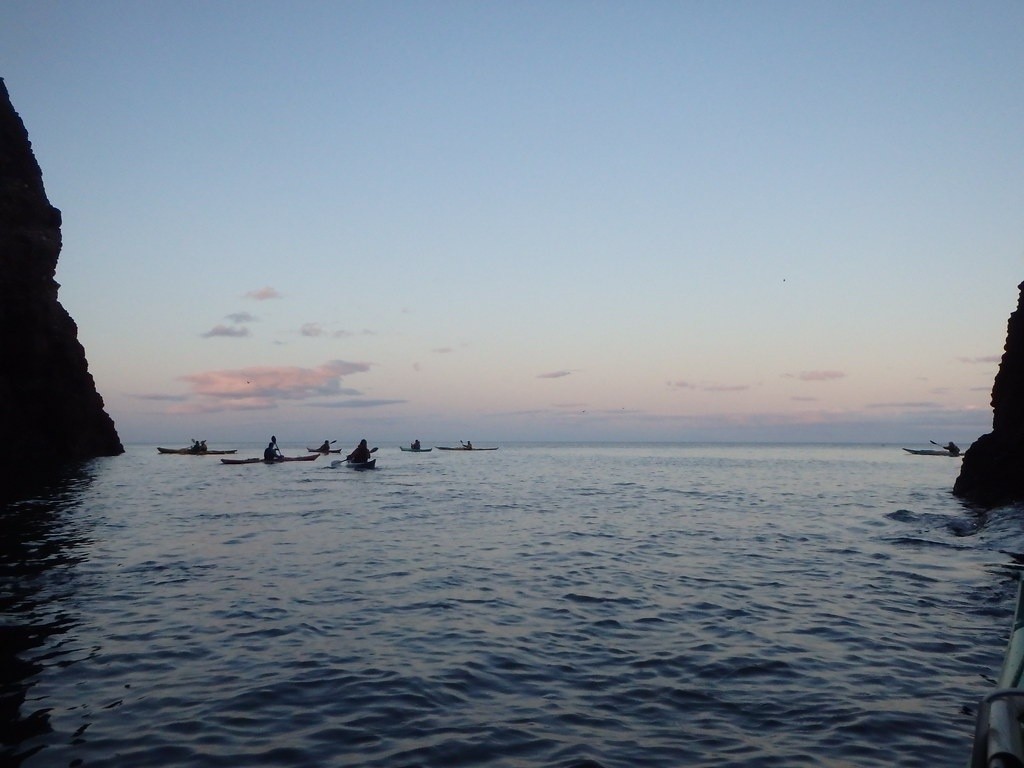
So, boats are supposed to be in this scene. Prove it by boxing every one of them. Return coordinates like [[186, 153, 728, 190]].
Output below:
[[400, 445, 432, 452], [345, 458, 376, 470], [221, 453, 321, 463], [902, 447, 965, 457], [157, 446, 237, 454], [306, 447, 342, 453], [434, 445, 500, 451]]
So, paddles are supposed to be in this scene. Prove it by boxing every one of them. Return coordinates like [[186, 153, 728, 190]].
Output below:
[[271, 435, 283, 462], [331, 447, 379, 467], [930, 440, 944, 448], [460, 440, 464, 445], [191, 439, 196, 445], [329, 439, 337, 445]]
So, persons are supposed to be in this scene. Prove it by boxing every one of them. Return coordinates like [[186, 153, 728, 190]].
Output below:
[[264, 442, 283, 461], [189, 441, 207, 452], [411, 440, 420, 450], [463, 441, 472, 450], [943, 441, 960, 454], [346, 438, 371, 463], [316, 440, 330, 452]]
